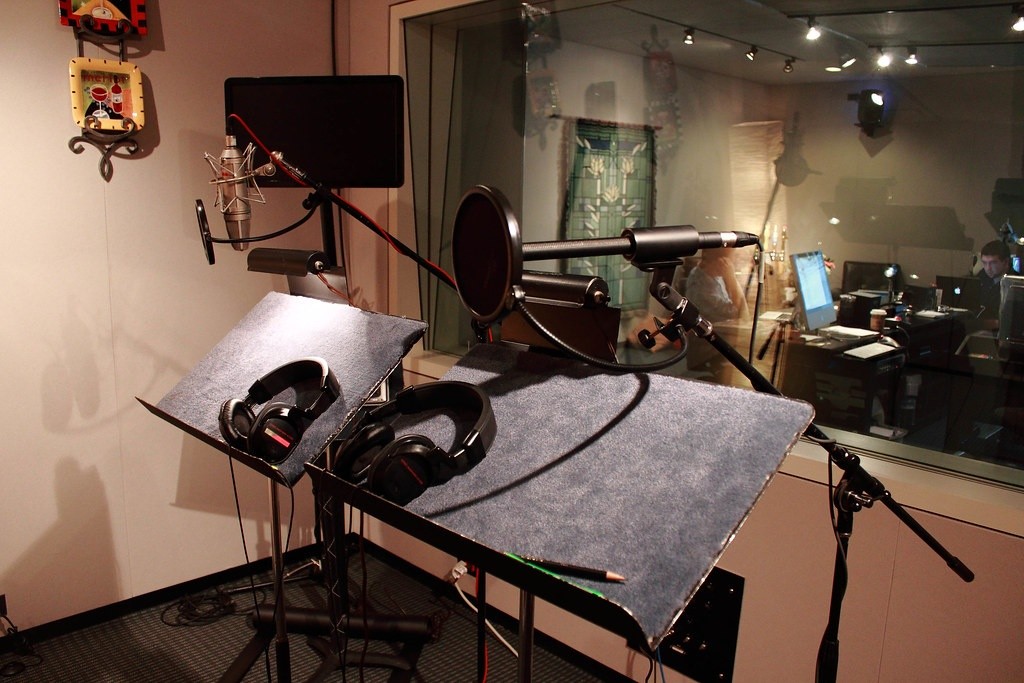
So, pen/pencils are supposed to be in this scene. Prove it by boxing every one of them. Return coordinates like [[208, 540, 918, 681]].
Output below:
[[504, 553, 627, 583], [830, 336, 843, 342]]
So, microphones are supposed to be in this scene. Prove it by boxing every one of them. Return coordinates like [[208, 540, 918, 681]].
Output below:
[[219, 125, 252, 252]]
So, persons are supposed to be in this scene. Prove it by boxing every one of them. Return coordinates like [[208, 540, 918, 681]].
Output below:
[[966, 241, 1021, 324], [686, 248, 749, 321]]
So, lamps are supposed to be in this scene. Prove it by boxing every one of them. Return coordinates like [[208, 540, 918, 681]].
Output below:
[[905, 47, 918, 65], [1013, 3, 1024, 32], [745, 45, 758, 62], [848, 89, 885, 138], [783, 57, 796, 73], [838, 43, 856, 68], [805, 15, 821, 41], [683, 27, 695, 45]]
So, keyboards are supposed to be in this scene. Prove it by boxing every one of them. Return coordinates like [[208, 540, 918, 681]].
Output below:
[[844, 342, 895, 359]]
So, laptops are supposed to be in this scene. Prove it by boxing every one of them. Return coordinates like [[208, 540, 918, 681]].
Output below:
[[935, 275, 983, 309]]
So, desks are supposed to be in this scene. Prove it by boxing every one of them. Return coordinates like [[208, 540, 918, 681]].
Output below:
[[685, 290, 992, 435]]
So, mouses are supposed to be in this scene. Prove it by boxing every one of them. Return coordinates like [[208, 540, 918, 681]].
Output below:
[[877, 335, 901, 347]]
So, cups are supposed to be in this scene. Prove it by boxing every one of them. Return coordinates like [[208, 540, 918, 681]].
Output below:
[[870, 309, 887, 332], [936, 289, 943, 307]]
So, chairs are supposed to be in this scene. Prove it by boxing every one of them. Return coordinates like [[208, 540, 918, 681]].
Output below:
[[841, 260, 905, 295]]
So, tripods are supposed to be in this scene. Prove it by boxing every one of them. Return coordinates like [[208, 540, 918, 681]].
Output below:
[[305, 496, 413, 683]]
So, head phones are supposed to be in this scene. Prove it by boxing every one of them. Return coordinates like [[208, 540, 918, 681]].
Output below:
[[334, 380, 497, 505], [218, 357, 341, 465]]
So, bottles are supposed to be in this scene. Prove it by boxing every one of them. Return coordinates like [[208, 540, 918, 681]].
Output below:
[[904, 304, 913, 323]]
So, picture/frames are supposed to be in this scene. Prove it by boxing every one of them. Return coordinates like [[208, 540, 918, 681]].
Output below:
[[58, 0, 148, 37], [70, 56, 145, 132]]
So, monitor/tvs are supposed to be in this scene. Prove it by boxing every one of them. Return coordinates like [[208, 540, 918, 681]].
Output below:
[[789, 250, 836, 331]]
